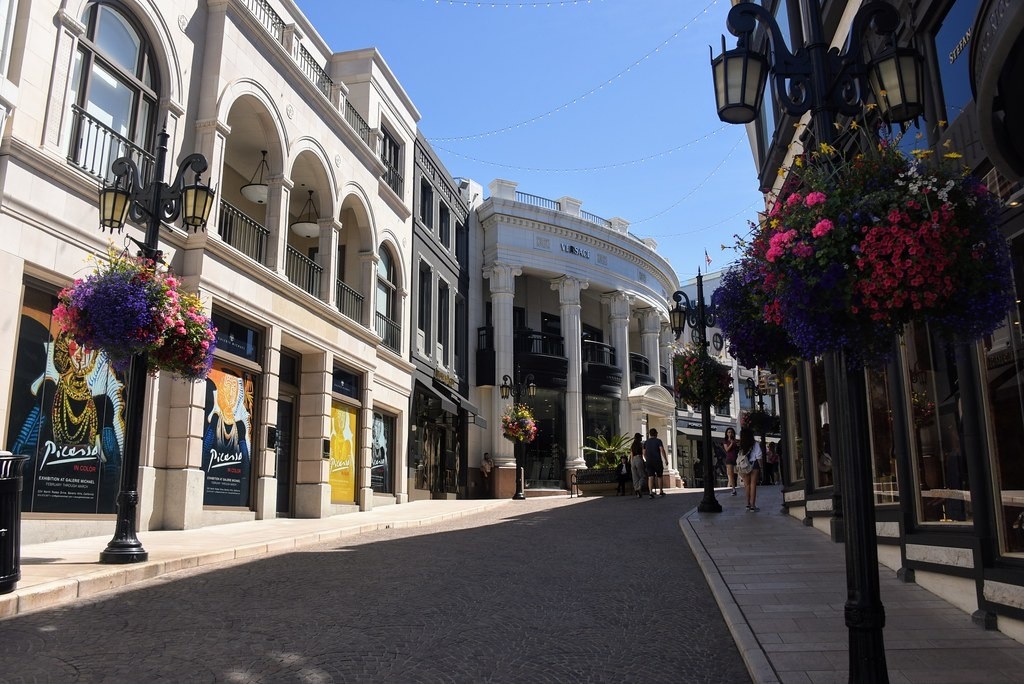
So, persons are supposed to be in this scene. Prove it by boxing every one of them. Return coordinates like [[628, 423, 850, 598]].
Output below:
[[643, 428, 668, 499], [820, 424, 832, 484], [740, 428, 763, 512], [722, 427, 740, 496], [616, 455, 632, 496], [767, 440, 782, 485], [480, 452, 494, 498], [631, 432, 645, 498]]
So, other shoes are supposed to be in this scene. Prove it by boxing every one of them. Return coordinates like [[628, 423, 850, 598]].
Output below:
[[635, 489, 641, 498], [745, 505, 761, 513], [649, 494, 654, 499], [659, 492, 666, 497], [731, 492, 736, 496], [621, 493, 625, 496]]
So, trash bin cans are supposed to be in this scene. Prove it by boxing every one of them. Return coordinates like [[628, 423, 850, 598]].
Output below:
[[0, 454, 31, 595]]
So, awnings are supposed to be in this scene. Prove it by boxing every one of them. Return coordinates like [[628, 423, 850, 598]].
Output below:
[[677, 427, 780, 443], [417, 379, 487, 429]]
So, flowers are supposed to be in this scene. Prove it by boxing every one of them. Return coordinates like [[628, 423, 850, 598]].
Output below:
[[52, 242, 217, 381], [708, 88, 1019, 375], [667, 342, 735, 410], [502, 405, 538, 444], [909, 390, 935, 428], [740, 410, 772, 435]]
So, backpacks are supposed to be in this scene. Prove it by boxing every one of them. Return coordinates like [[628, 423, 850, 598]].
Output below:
[[736, 445, 753, 475]]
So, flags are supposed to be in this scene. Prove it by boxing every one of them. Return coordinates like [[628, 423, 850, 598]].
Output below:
[[705, 251, 712, 265]]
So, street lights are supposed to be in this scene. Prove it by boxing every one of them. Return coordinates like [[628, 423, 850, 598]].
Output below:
[[668, 265, 732, 513], [96, 115, 218, 565], [500, 362, 537, 500], [707, 0, 929, 684], [745, 377, 776, 485]]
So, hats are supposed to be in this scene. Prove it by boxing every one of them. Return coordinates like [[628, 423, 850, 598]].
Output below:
[[634, 433, 643, 437], [620, 455, 628, 461]]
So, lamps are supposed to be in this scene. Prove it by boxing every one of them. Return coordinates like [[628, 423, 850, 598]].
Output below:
[[290, 190, 321, 239], [239, 150, 272, 204]]
